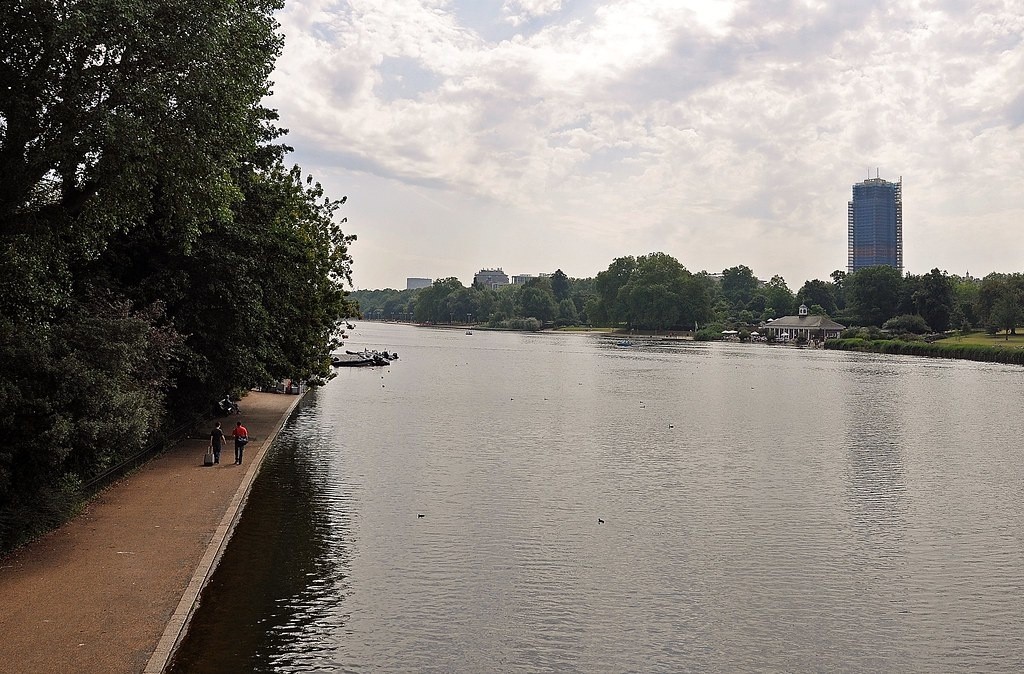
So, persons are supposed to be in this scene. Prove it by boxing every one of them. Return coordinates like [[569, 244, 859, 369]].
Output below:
[[233, 422, 248, 465], [225, 395, 242, 415], [211, 422, 226, 464]]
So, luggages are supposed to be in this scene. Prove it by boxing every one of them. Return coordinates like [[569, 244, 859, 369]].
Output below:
[[204, 445, 214, 466]]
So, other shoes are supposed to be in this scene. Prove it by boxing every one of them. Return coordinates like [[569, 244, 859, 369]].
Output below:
[[214, 461, 219, 464], [235, 461, 238, 464], [239, 462, 241, 465]]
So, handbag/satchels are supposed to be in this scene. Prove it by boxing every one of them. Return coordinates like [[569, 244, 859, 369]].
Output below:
[[235, 436, 248, 446]]
